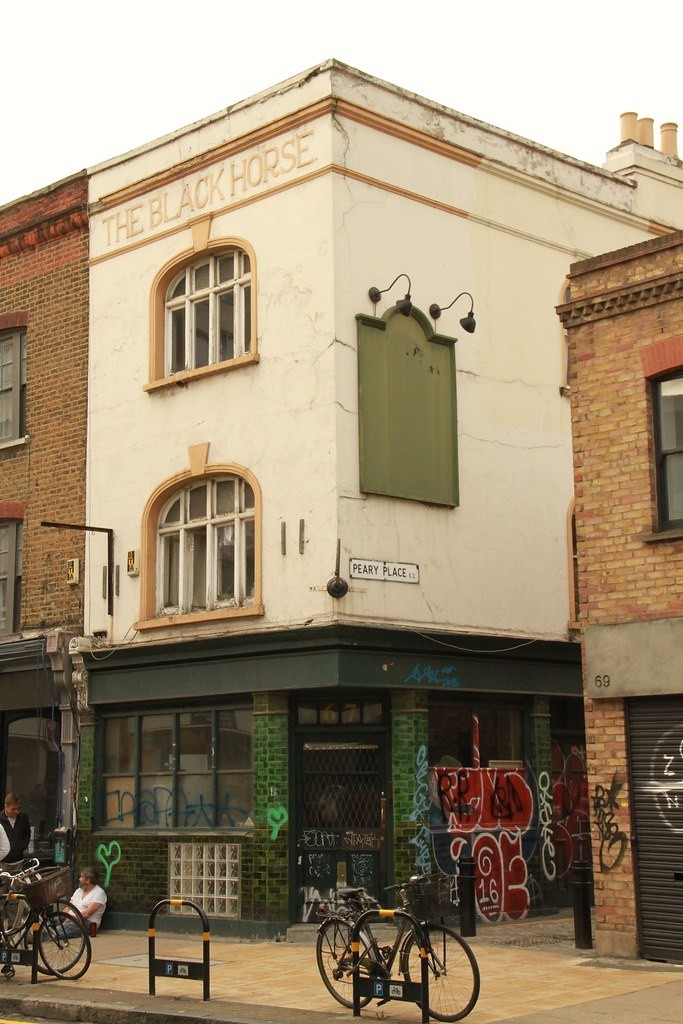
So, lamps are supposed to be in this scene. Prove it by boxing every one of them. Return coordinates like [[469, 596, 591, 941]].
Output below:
[[329, 538, 348, 599], [374, 298, 375, 299], [369, 273, 412, 317], [429, 292, 475, 334]]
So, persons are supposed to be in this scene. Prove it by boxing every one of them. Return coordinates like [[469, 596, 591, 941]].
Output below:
[[21, 869, 107, 944], [0, 793, 31, 931], [0, 824, 10, 860]]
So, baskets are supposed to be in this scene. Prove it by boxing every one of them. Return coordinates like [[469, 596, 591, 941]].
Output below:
[[405, 873, 452, 920], [18, 866, 73, 910]]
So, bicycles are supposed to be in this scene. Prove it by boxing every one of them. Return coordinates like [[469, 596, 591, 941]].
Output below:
[[1, 858, 92, 981], [300, 870, 482, 1024]]
